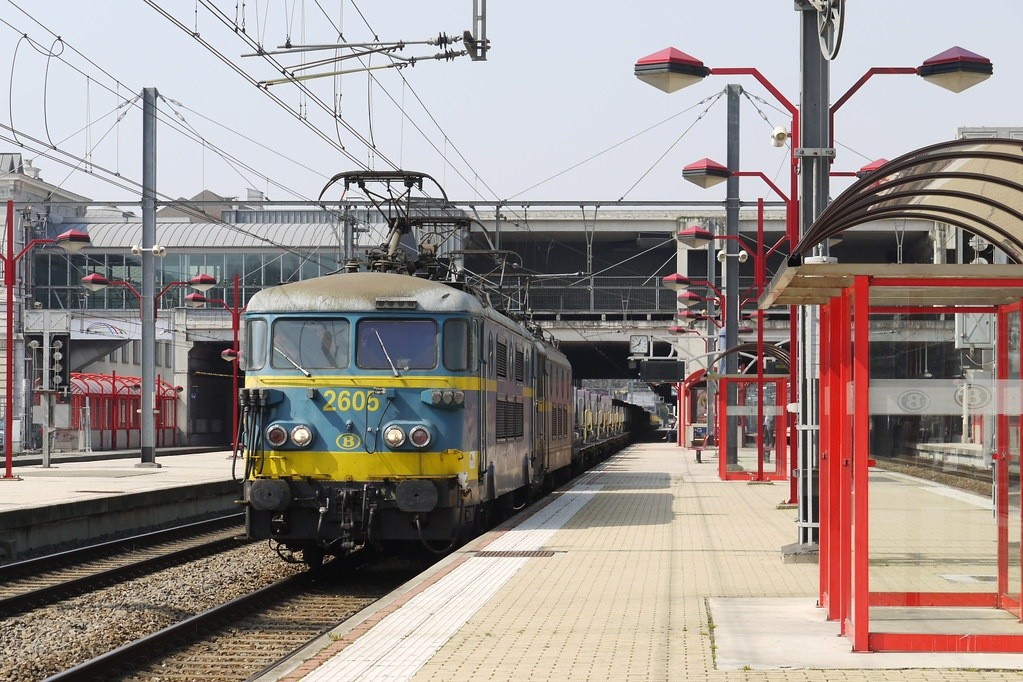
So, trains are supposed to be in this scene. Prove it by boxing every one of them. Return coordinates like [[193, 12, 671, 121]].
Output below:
[[232, 264, 660, 578]]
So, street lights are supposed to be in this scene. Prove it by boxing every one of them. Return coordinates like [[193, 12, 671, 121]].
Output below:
[[631, 42, 995, 182], [187, 288, 245, 461], [679, 155, 894, 256], [664, 227, 801, 487], [80, 265, 216, 320], [0, 198, 90, 479]]
